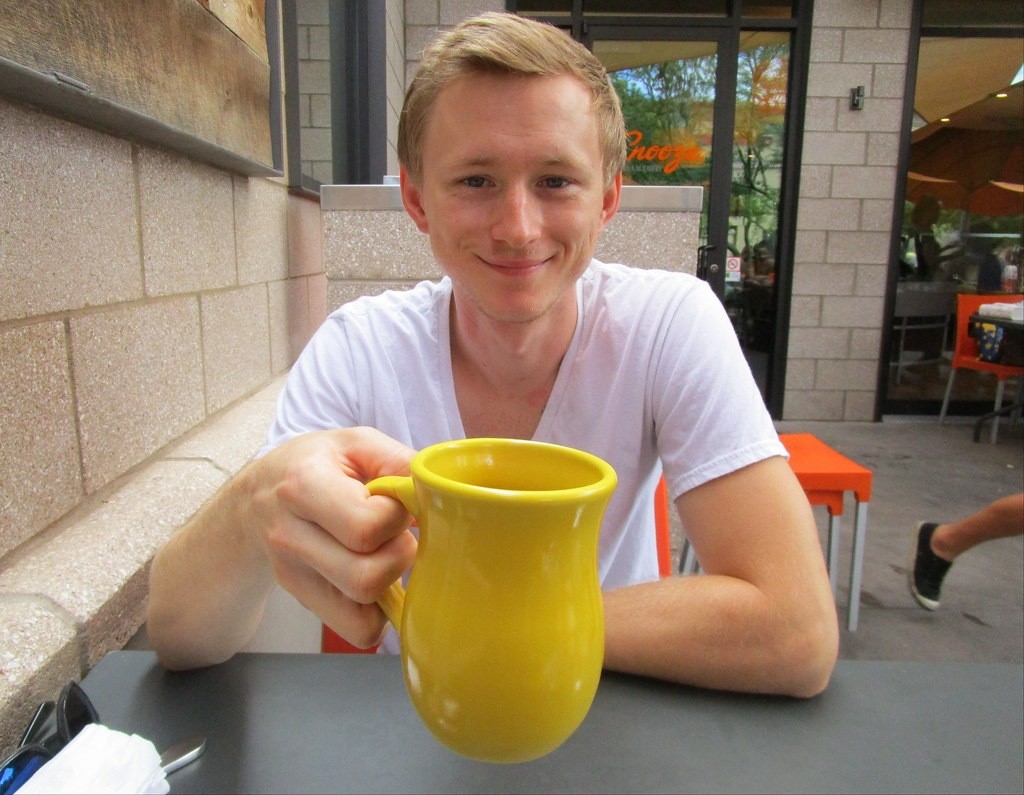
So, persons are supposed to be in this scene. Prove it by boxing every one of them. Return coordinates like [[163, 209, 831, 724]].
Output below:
[[743, 195, 1024, 360], [907, 491, 1024, 611], [146, 9, 841, 697]]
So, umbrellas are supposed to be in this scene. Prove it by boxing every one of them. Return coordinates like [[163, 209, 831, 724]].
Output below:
[[903, 80, 1024, 217]]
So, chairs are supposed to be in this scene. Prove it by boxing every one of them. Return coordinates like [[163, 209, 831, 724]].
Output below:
[[938, 293, 1024, 445]]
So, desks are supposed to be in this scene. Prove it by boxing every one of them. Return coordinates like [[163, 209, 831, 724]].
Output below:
[[968, 312, 1024, 441], [889, 283, 952, 387], [79, 650, 1024, 795]]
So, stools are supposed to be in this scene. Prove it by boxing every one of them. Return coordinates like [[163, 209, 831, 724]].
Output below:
[[678, 433, 871, 632]]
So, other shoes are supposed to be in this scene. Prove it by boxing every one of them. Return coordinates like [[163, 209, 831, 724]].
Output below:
[[911, 520, 953, 610]]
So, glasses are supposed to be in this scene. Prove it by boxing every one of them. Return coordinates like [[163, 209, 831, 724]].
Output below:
[[0, 681, 101, 795]]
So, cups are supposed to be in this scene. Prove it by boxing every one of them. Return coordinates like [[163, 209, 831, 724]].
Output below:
[[1000, 264, 1018, 295], [365, 438, 618, 765]]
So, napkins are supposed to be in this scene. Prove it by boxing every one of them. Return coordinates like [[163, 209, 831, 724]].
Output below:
[[10, 722, 171, 795]]
